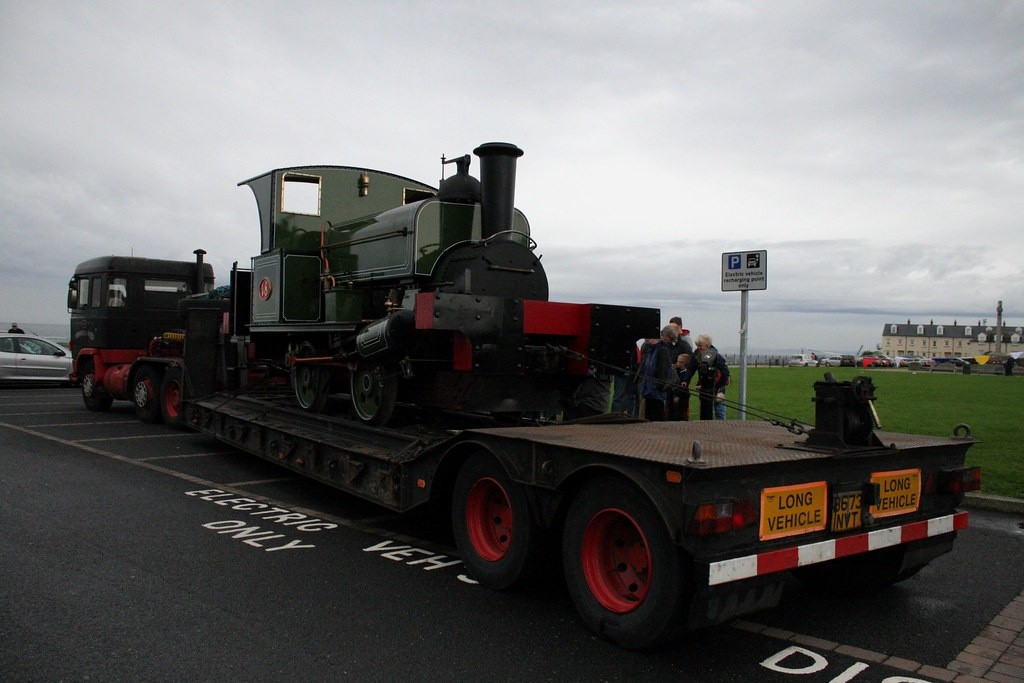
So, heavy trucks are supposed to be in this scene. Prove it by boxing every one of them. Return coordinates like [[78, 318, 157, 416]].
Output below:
[[68, 256, 985, 653]]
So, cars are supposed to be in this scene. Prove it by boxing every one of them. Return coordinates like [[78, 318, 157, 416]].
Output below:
[[931, 357, 970, 368], [821, 356, 841, 367], [860, 354, 894, 367], [840, 355, 859, 367], [0, 332, 73, 384], [899, 355, 930, 367], [788, 354, 820, 367]]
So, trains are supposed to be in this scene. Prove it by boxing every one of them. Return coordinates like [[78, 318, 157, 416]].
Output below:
[[177, 142, 661, 426]]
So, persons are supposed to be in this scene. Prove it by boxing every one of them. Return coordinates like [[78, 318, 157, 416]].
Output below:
[[573, 316, 731, 421], [7, 323, 25, 347]]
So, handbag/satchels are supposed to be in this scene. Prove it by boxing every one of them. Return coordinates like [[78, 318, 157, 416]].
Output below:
[[714, 357, 730, 386]]
